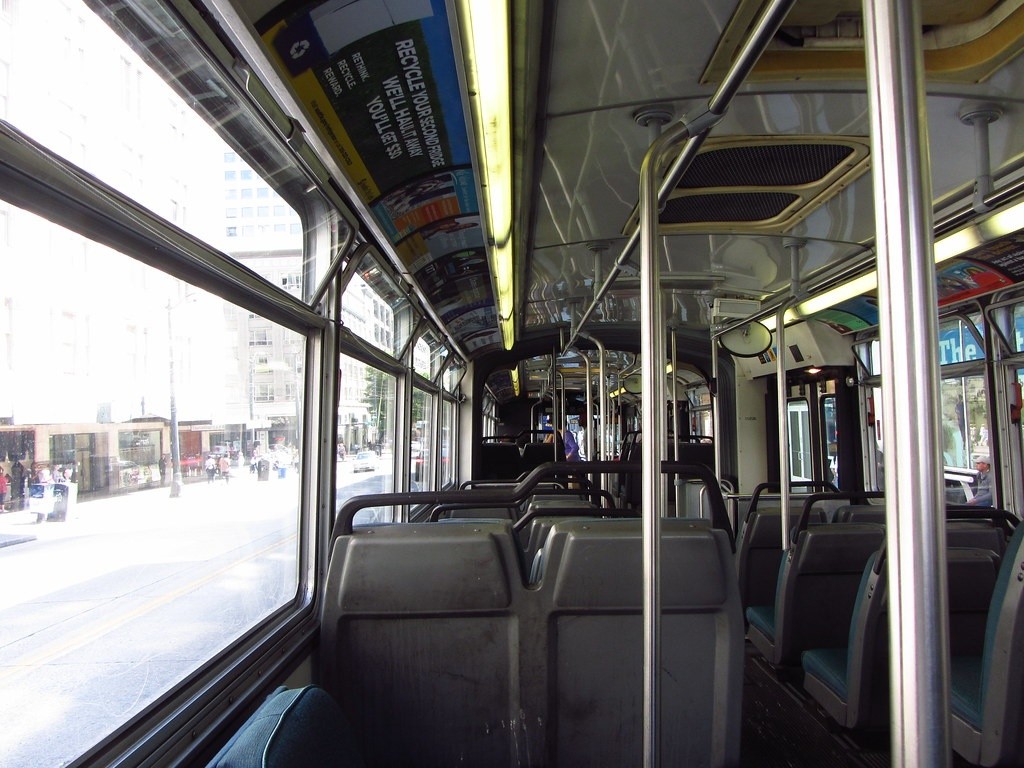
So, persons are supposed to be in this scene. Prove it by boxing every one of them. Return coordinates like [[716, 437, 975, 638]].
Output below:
[[249, 440, 345, 473], [355, 443, 358, 454], [965, 456, 992, 507], [158, 450, 230, 484], [501, 417, 581, 490], [0, 455, 27, 513]]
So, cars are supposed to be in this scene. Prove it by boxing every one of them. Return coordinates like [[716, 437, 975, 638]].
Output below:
[[415, 448, 448, 480], [353, 451, 378, 472]]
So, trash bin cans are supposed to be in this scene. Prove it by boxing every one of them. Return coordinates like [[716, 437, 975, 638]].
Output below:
[[258, 460, 270, 481], [48, 483, 78, 522], [374, 445, 382, 456]]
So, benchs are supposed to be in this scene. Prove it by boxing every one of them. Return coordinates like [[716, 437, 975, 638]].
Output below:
[[204, 429, 1024, 768]]
